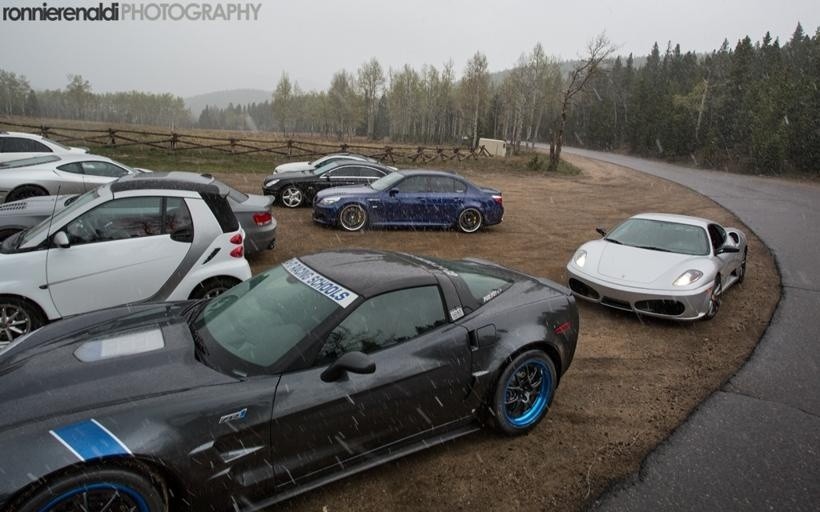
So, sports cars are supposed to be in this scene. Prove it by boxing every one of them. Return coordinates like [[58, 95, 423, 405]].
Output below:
[[1, 249, 579, 511], [567, 212, 749, 322]]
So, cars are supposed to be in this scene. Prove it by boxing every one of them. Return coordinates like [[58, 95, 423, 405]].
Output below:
[[274, 153, 378, 174], [1, 170, 278, 257], [313, 170, 505, 233], [0, 153, 147, 204], [0, 181, 252, 344], [260, 162, 400, 207], [0, 130, 90, 164]]
